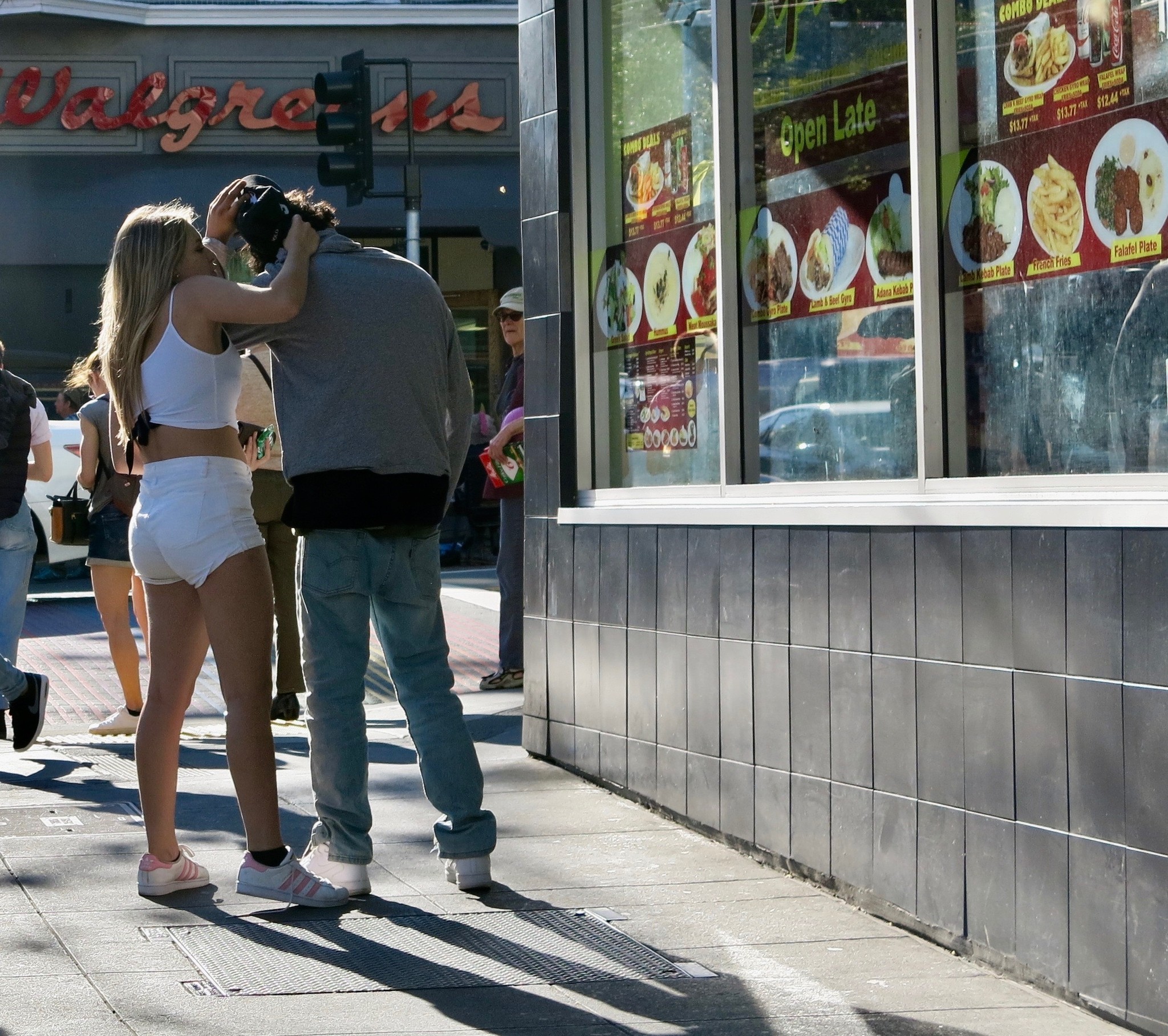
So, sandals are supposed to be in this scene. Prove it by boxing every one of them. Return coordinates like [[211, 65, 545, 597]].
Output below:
[[479, 666, 523, 690]]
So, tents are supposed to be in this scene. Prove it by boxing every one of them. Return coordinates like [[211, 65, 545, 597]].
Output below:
[[479, 441, 525, 488]]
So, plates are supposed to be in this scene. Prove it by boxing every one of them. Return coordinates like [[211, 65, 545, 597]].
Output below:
[[685, 380, 693, 398], [1027, 163, 1084, 258], [1085, 118, 1168, 250], [661, 428, 679, 447], [865, 193, 913, 285], [688, 400, 696, 418], [688, 420, 696, 447], [596, 266, 642, 339], [742, 221, 798, 310], [682, 230, 717, 319], [948, 160, 1023, 273], [640, 407, 660, 423], [799, 224, 865, 300], [679, 429, 688, 447], [644, 242, 681, 331], [644, 429, 661, 448]]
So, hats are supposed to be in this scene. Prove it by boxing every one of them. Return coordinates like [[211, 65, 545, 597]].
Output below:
[[63, 387, 83, 408], [235, 174, 292, 254], [491, 286, 523, 313]]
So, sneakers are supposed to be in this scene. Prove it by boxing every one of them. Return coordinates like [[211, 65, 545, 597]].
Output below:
[[299, 839, 370, 895], [444, 854, 491, 889], [8, 672, 49, 752], [138, 843, 210, 895], [234, 845, 350, 907], [89, 704, 140, 736]]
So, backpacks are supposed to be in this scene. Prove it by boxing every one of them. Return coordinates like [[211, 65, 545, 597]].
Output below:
[[96, 398, 142, 516]]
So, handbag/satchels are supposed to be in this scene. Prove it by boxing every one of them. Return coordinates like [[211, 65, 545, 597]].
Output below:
[[47, 481, 92, 546]]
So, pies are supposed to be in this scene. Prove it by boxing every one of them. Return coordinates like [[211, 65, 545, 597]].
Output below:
[[1010, 29, 1039, 74], [628, 163, 645, 195], [805, 233, 834, 296]]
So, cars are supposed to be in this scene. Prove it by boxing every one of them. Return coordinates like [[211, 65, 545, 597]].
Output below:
[[752, 356, 923, 482], [26, 420, 101, 566]]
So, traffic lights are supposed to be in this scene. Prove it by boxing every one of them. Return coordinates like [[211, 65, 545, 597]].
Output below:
[[315, 48, 372, 208]]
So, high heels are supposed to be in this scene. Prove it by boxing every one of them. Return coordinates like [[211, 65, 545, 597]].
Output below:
[[270, 692, 300, 720]]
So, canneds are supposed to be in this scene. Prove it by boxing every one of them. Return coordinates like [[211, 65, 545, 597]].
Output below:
[[663, 137, 688, 195], [1076, 0, 1124, 68]]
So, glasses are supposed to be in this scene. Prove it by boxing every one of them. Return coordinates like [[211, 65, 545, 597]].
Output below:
[[495, 311, 523, 322], [87, 386, 95, 399]]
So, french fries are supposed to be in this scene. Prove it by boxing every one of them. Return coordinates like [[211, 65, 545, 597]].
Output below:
[[638, 161, 661, 203], [1033, 154, 1080, 257], [1032, 25, 1070, 84]]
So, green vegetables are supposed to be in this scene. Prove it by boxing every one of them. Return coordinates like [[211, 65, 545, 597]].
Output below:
[[749, 234, 767, 272], [694, 240, 715, 283], [653, 250, 670, 304], [869, 202, 902, 257], [963, 163, 1009, 225], [603, 252, 636, 331], [1094, 156, 1121, 231]]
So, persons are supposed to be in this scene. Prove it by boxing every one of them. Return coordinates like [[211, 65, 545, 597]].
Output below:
[[0, 343, 54, 750], [65, 353, 151, 734], [55, 388, 83, 421], [93, 206, 348, 908], [201, 175, 496, 895], [1111, 262, 1168, 473], [234, 344, 305, 722], [480, 288, 524, 688]]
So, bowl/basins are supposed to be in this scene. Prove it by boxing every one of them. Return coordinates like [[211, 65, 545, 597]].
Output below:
[[1004, 31, 1076, 97], [626, 167, 664, 212], [660, 412, 670, 422]]
